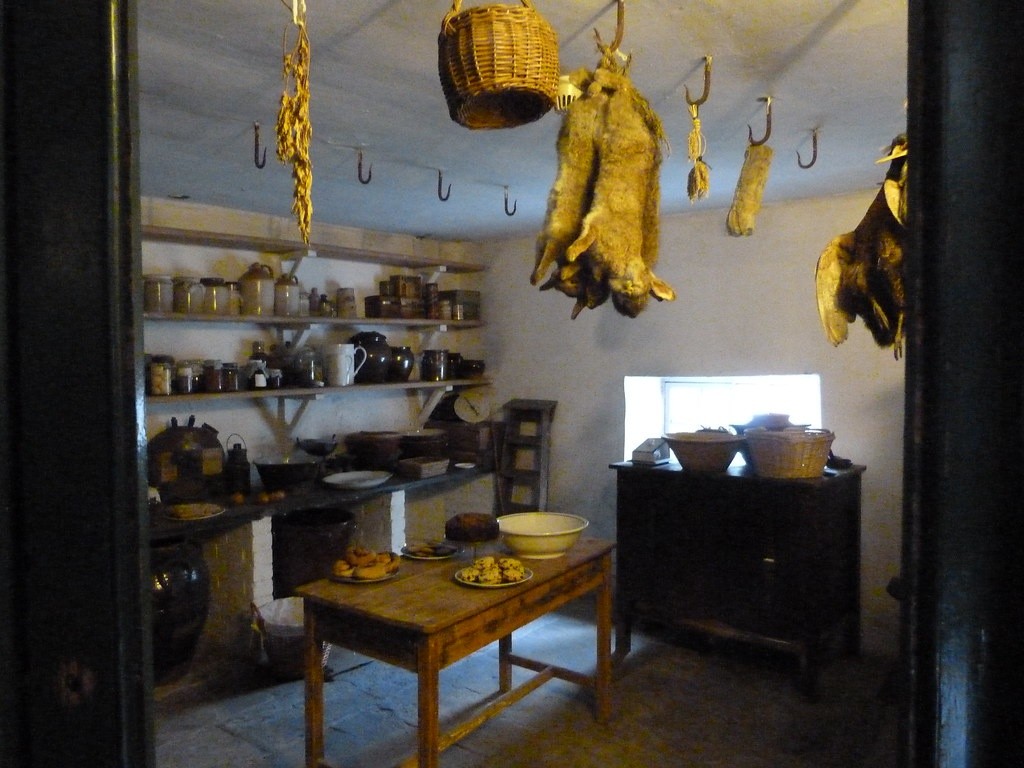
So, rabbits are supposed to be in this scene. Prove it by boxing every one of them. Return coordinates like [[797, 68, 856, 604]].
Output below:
[[528, 47, 677, 324]]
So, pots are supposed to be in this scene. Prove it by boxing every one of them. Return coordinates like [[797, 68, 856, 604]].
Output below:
[[252, 452, 319, 494]]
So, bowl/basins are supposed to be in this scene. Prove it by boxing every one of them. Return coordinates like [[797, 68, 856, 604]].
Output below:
[[498, 512, 590, 559], [402, 429, 450, 458], [345, 430, 400, 467], [463, 359, 483, 377], [662, 432, 745, 472]]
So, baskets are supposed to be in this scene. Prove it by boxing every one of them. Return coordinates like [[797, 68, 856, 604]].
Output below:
[[436, 0, 559, 130], [743, 427, 835, 478], [398, 456, 449, 479]]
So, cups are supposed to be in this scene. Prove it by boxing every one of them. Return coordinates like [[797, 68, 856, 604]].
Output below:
[[322, 341, 367, 387]]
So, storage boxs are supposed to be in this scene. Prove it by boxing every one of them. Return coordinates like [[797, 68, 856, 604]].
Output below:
[[365, 275, 482, 320]]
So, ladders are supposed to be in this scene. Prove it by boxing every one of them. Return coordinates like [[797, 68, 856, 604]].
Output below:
[[500, 399, 558, 513]]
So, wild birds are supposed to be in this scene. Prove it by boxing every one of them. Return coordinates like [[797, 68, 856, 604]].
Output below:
[[817, 134, 908, 361]]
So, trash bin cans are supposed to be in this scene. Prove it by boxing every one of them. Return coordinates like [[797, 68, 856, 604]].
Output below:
[[273, 508, 356, 599]]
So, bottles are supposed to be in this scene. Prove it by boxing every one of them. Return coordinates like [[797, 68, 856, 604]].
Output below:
[[148, 539, 210, 686], [145, 262, 333, 318], [349, 331, 391, 383], [226, 444, 251, 495], [423, 282, 464, 320], [145, 339, 319, 396], [421, 349, 449, 381], [446, 352, 463, 378], [388, 346, 414, 382]]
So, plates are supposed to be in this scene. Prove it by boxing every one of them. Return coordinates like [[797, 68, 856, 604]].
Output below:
[[455, 567, 533, 587], [401, 546, 457, 560], [328, 567, 401, 582], [455, 463, 475, 469], [322, 470, 394, 490], [161, 503, 226, 520]]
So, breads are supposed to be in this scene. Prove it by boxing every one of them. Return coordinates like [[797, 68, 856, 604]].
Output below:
[[334, 545, 401, 579], [458, 556, 524, 585]]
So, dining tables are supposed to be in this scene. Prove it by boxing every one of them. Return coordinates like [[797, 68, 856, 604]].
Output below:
[[295, 536, 618, 768]]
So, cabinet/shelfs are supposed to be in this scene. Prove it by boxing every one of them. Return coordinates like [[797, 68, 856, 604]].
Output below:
[[608, 456, 867, 706], [141, 223, 494, 449]]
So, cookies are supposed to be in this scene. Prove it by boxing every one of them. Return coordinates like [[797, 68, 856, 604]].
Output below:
[[405, 539, 459, 557]]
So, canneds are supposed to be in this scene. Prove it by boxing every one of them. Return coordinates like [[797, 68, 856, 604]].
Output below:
[[452, 303, 463, 321], [424, 282, 440, 319], [336, 287, 356, 319]]
